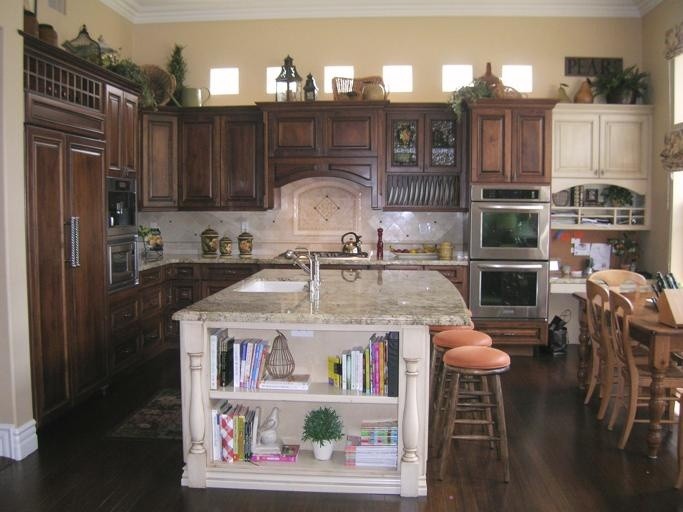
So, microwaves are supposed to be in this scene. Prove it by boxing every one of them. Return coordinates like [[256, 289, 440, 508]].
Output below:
[[104, 235, 137, 287], [107, 177, 138, 232]]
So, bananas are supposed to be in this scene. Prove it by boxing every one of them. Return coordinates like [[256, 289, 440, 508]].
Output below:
[[424, 244, 437, 253]]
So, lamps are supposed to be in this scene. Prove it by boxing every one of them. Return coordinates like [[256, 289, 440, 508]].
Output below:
[[302, 72, 319, 101], [273, 54, 305, 102]]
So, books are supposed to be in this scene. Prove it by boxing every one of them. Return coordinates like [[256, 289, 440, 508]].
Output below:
[[324, 330, 398, 396], [345, 417, 398, 473], [210, 326, 271, 391], [211, 400, 259, 463], [260, 374, 312, 391], [251, 444, 302, 462]]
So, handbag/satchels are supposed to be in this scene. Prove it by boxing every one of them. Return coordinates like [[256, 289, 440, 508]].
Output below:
[[548, 316, 569, 357]]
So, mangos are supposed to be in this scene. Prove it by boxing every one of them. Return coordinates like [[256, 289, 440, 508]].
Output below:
[[409, 249, 416, 253]]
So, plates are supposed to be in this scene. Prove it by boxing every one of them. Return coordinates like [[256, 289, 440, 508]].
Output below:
[[391, 251, 440, 257]]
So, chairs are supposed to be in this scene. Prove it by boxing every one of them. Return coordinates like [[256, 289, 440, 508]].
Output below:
[[609, 290, 683, 449], [587, 270, 650, 291], [583, 280, 650, 420]]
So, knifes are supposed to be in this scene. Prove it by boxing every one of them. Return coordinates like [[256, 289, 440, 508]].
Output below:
[[652, 271, 677, 297]]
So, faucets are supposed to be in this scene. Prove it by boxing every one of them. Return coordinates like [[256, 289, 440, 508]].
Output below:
[[293, 253, 321, 293]]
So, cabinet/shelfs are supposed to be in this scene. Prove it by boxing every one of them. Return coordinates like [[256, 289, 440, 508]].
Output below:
[[105, 83, 141, 180], [465, 98, 560, 184], [552, 103, 656, 178], [24, 121, 111, 432], [551, 178, 648, 294], [255, 101, 390, 210], [107, 263, 255, 369], [384, 264, 469, 322], [22, 34, 107, 140], [382, 103, 471, 211], [177, 320, 433, 499], [181, 106, 264, 211], [469, 320, 549, 357], [137, 107, 182, 211]]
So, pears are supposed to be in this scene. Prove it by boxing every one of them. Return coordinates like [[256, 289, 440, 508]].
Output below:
[[573, 82, 593, 104], [554, 86, 571, 102]]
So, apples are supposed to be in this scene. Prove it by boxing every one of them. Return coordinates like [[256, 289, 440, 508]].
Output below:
[[402, 249, 409, 253], [396, 248, 401, 253]]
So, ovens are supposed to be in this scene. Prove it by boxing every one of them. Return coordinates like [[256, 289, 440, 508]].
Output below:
[[469, 183, 550, 320]]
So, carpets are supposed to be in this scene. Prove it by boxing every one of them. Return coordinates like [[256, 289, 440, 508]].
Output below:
[[107, 385, 182, 441]]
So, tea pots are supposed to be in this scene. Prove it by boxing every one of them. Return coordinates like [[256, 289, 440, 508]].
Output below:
[[341, 232, 362, 254]]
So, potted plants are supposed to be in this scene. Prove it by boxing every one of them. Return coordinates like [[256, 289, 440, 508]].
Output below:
[[586, 60, 650, 104], [164, 44, 186, 106], [300, 404, 346, 462]]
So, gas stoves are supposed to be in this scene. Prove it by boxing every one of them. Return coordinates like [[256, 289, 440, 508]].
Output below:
[[274, 247, 372, 260]]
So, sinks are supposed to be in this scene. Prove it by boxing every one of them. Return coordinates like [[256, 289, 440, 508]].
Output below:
[[233, 280, 310, 294]]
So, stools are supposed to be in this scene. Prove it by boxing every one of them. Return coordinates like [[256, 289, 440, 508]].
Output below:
[[437, 346, 512, 482], [430, 324, 480, 411], [431, 330, 496, 450]]
